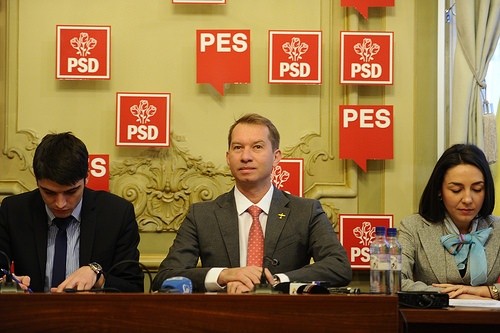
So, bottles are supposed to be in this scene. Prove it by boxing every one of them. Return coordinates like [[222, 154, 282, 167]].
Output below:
[[369, 226, 401, 293]]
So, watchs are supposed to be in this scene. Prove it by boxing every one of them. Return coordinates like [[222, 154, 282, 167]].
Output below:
[[87, 262, 102, 283]]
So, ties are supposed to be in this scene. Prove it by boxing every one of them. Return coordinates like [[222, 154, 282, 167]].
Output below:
[[246, 206, 264, 267], [50, 215, 74, 288]]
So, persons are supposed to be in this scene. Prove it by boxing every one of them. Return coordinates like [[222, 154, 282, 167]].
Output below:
[[0, 131, 140, 292], [380, 144, 500, 300], [152, 115, 351, 294]]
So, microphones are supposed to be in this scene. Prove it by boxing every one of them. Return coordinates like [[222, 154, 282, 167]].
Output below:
[[157, 276, 192, 294], [64, 287, 122, 293]]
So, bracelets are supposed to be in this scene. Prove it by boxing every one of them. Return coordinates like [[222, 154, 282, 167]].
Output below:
[[272, 275, 278, 287], [487, 286, 492, 298]]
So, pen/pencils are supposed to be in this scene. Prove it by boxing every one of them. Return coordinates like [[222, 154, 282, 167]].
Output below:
[[327, 287, 361, 293], [0, 269, 34, 294]]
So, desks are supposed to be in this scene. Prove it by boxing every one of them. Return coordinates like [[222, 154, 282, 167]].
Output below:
[[0, 292, 500, 333]]
